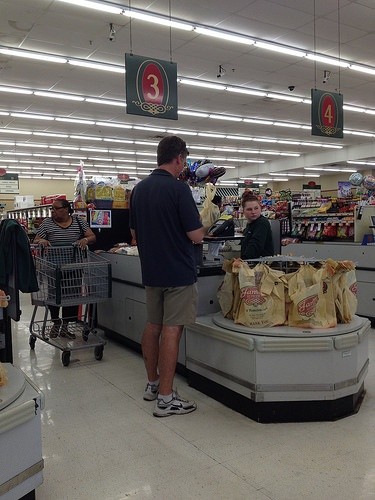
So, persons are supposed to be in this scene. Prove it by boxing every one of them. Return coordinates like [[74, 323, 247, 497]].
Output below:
[[129, 136, 205, 417], [33, 199, 97, 339], [211, 196, 222, 209], [238, 195, 274, 264]]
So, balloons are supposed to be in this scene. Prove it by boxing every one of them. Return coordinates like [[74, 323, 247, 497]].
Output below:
[[349, 172, 364, 187], [363, 175, 375, 191]]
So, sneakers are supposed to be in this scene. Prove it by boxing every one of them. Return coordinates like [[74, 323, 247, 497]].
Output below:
[[143, 383, 160, 401], [153, 390, 197, 417]]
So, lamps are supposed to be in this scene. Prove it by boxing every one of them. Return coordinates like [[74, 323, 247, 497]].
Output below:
[[0, 0, 375, 187]]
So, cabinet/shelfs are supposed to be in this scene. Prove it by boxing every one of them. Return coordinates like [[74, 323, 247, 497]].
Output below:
[[7, 201, 75, 243], [224, 196, 375, 244]]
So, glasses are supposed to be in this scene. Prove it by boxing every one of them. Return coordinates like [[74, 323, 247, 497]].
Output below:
[[50, 207, 67, 212]]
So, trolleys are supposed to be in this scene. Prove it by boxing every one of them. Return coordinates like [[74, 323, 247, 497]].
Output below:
[[29, 244, 112, 366]]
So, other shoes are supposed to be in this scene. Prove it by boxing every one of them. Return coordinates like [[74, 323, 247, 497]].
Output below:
[[50, 321, 61, 338], [60, 330, 76, 339]]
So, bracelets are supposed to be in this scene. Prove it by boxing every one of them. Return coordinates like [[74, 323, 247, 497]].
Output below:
[[84, 238, 88, 242]]
